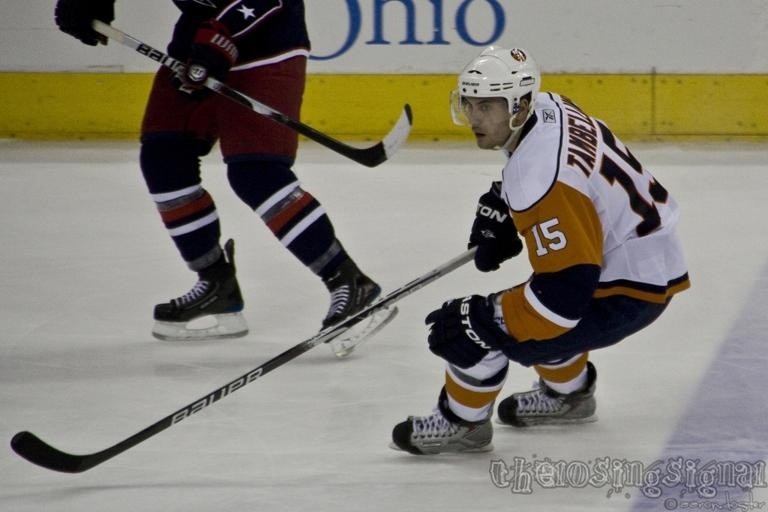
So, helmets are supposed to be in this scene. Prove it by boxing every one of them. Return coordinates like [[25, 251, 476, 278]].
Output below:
[[449, 45, 541, 132]]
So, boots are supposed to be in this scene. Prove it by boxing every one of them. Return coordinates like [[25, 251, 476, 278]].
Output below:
[[320, 238, 380, 343], [393, 385, 495, 456], [497, 361, 597, 427], [154, 238, 244, 322]]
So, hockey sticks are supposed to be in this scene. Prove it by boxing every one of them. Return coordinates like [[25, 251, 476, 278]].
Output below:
[[92, 18, 413, 167], [11, 246, 478, 474]]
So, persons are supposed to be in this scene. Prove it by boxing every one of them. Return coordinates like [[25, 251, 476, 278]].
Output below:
[[393, 45, 690, 455], [56, 1, 381, 345]]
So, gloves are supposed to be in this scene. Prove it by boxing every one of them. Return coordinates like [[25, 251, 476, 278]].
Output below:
[[55, 0, 115, 46], [425, 293, 519, 369], [467, 181, 523, 272], [168, 18, 240, 100]]
